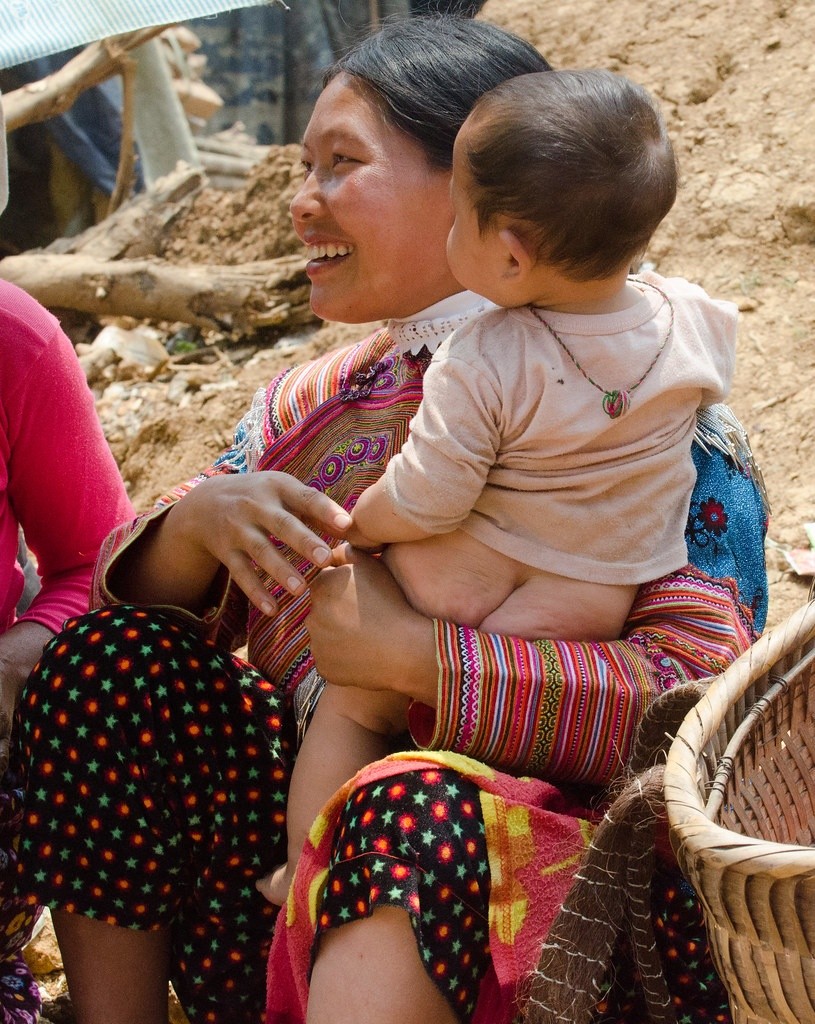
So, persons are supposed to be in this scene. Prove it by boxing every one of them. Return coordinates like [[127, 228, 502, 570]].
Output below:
[[256, 71, 741, 905], [0, 277, 141, 1024], [0, 20, 772, 1024]]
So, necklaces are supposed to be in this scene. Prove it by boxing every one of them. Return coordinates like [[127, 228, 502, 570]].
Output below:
[[527, 276, 674, 420]]
